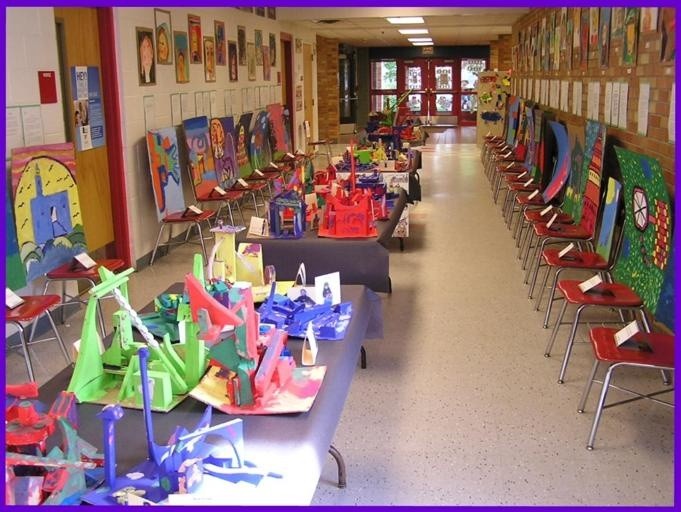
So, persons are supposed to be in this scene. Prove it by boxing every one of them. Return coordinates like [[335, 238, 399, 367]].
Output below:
[[230, 51, 238, 80], [204, 37, 215, 81], [178, 53, 188, 82], [140, 35, 156, 84]]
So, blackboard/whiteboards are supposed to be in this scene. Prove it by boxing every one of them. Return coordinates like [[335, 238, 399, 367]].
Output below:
[[4, 7, 68, 159]]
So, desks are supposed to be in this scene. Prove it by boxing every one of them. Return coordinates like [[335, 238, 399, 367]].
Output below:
[[367, 130, 430, 150], [235, 187, 413, 297], [6, 281, 384, 507], [332, 147, 422, 201]]
